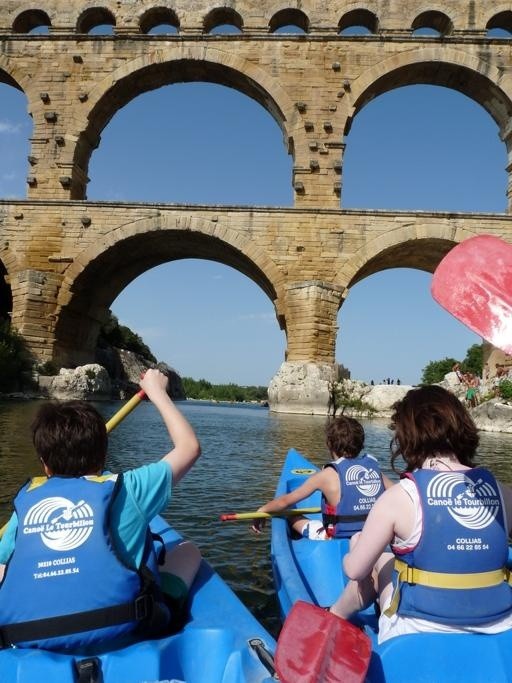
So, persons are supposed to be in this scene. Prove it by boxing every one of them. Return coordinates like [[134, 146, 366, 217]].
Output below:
[[251, 417, 396, 542], [328, 386, 512, 642], [0, 368, 201, 649], [369, 377, 402, 385], [483, 362, 509, 380], [451, 361, 481, 408]]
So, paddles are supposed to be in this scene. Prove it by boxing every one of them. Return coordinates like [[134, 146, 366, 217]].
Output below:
[[430, 234, 512, 358], [272, 599, 372, 683]]
